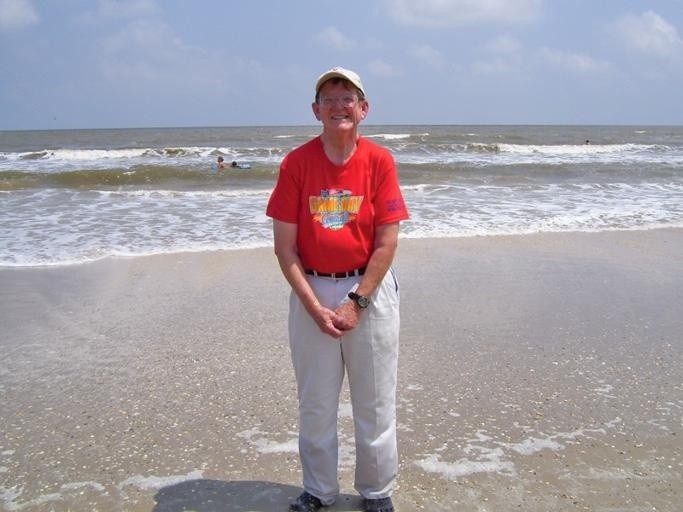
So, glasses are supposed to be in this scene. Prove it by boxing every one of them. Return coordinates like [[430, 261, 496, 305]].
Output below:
[[320, 95, 357, 107]]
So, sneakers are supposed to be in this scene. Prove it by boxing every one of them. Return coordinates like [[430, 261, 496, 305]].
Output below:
[[289, 492, 321, 512], [365, 497, 395, 511]]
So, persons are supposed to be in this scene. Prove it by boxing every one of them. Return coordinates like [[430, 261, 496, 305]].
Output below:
[[230, 161, 240, 168], [264, 66, 411, 512], [217, 156, 226, 168]]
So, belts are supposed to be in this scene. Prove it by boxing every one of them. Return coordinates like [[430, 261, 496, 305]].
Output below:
[[304, 268, 366, 279]]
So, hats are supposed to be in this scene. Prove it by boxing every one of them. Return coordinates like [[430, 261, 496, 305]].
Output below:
[[315, 66, 365, 95]]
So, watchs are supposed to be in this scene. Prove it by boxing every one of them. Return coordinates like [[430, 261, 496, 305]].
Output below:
[[348, 292, 371, 308]]
